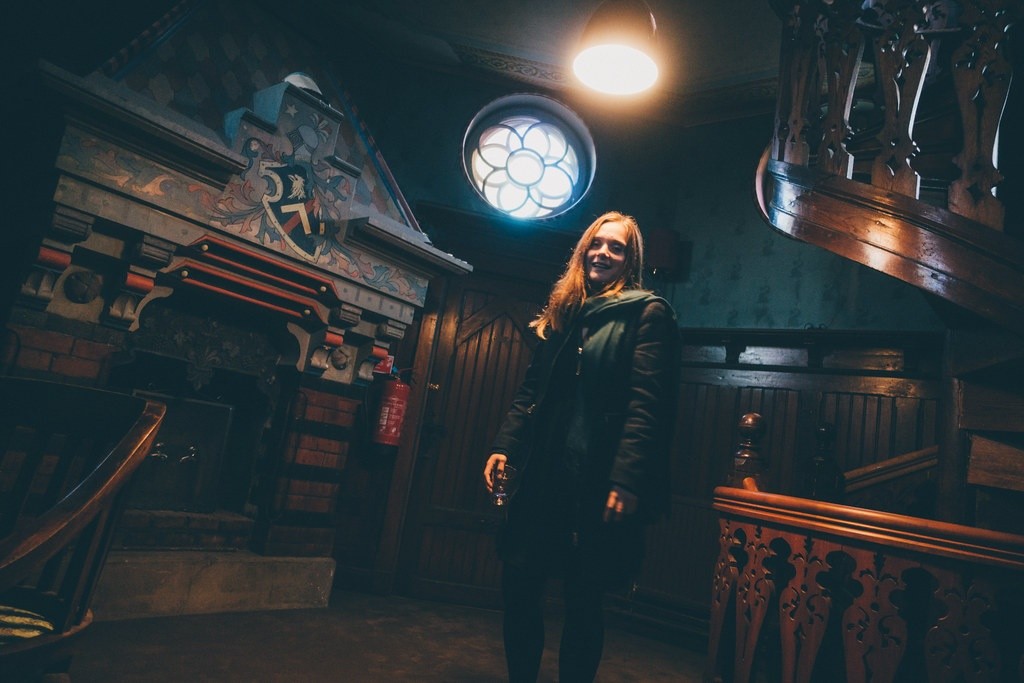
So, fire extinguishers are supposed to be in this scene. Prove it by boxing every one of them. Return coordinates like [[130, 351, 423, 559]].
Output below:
[[373, 366, 418, 448]]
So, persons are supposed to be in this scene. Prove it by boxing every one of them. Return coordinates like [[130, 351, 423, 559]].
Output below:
[[484, 212, 684, 683]]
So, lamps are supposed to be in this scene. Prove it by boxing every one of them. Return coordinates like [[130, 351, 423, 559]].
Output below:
[[573, 1, 665, 97]]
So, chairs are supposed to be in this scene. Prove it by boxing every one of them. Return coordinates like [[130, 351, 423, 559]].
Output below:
[[0, 370, 165, 683]]
